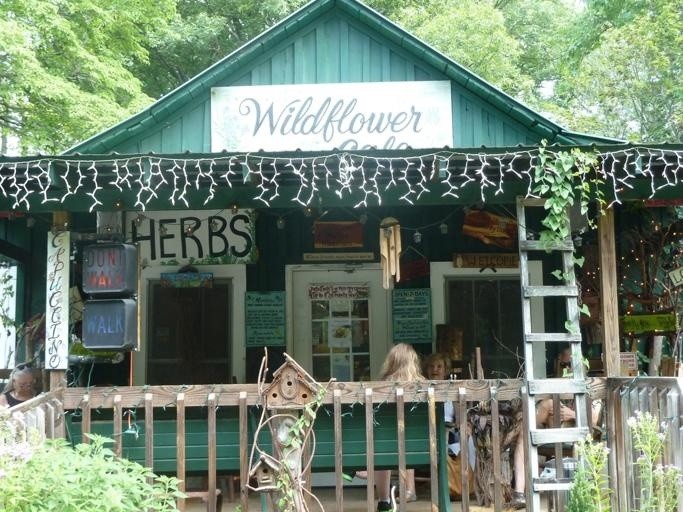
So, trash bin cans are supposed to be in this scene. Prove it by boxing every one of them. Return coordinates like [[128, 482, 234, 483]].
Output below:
[[542, 458, 581, 512]]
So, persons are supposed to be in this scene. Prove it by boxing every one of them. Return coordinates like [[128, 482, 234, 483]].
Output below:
[[496, 348, 602, 508], [0, 363, 44, 408], [340, 343, 424, 512], [392, 353, 473, 503]]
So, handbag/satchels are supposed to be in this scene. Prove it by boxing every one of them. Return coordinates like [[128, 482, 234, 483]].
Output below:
[[446, 448, 474, 496]]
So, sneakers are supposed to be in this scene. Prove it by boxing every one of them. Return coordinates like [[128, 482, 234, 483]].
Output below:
[[340, 469, 356, 481], [376, 499, 394, 512], [395, 491, 417, 503], [511, 491, 526, 508]]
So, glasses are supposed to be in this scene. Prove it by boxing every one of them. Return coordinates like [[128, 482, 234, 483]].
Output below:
[[16, 361, 33, 370], [559, 360, 572, 369]]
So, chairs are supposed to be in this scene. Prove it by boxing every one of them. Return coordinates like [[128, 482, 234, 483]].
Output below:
[[530, 365, 614, 471]]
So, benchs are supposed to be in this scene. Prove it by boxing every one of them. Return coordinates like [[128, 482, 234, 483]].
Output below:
[[66, 401, 453, 512]]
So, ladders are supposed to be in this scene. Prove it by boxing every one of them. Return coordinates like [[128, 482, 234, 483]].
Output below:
[[516, 194, 591, 512]]
